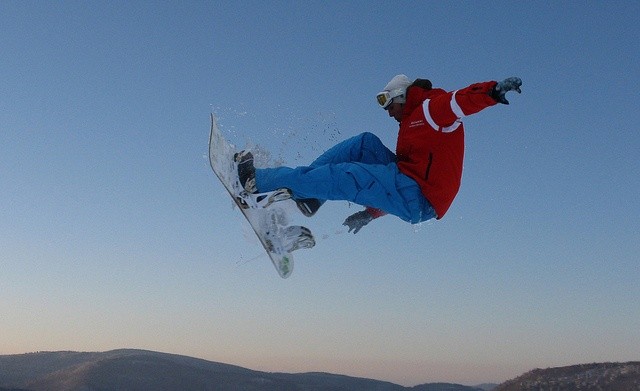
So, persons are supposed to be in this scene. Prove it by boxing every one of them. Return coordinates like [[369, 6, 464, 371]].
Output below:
[[233, 73, 524, 238]]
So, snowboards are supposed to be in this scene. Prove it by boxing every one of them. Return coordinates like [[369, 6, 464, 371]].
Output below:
[[208, 113, 295, 279]]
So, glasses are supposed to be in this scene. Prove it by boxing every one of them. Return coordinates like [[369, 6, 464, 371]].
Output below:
[[377, 88, 405, 108]]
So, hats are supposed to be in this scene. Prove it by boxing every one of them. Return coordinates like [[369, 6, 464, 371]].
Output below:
[[384, 75, 412, 103]]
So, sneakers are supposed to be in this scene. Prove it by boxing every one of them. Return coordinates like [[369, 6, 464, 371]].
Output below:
[[297, 198, 321, 217], [238, 150, 259, 194]]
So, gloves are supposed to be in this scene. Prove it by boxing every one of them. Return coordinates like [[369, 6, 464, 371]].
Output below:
[[491, 77, 522, 105], [342, 211, 373, 234]]
